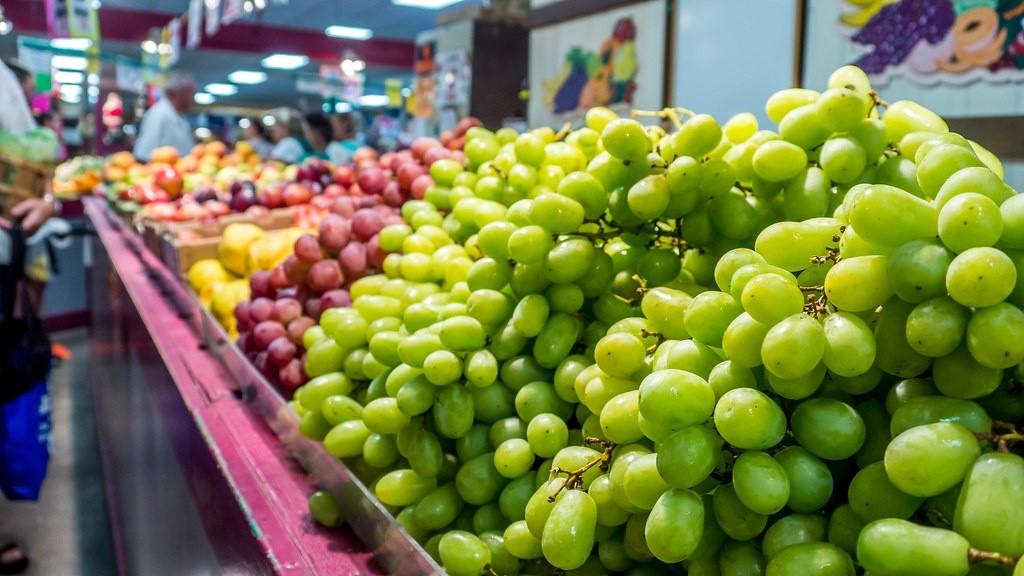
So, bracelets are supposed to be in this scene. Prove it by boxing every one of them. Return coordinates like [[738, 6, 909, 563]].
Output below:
[[44, 194, 64, 216]]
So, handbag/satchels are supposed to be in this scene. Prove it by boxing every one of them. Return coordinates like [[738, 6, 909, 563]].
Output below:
[[0, 215, 51, 501]]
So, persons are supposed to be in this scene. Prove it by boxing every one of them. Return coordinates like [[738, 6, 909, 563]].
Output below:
[[241, 107, 370, 169], [0, 58, 84, 575], [132, 72, 197, 165]]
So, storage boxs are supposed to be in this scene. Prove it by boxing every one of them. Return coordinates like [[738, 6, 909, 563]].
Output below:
[[146, 204, 295, 284]]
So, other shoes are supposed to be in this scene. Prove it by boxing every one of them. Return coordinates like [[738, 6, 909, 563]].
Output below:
[[0, 536, 29, 576]]
